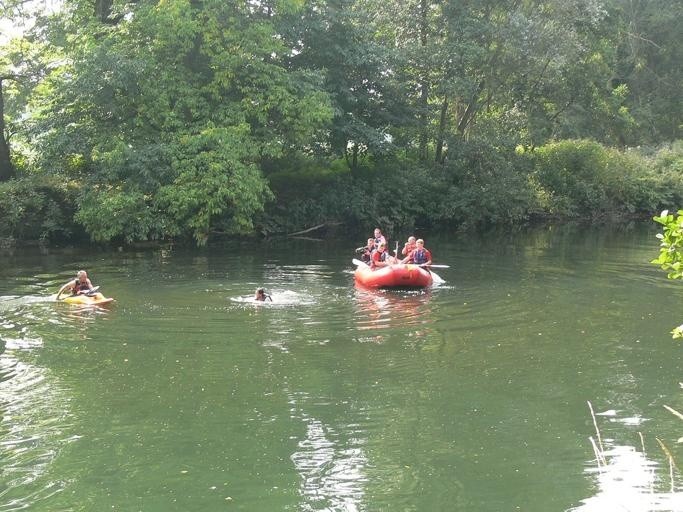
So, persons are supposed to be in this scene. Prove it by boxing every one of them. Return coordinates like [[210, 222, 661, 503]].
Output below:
[[56, 270, 93, 300], [402, 236, 417, 255], [370, 242, 390, 271], [361, 238, 375, 264], [400, 239, 432, 271], [254, 288, 272, 302], [373, 228, 386, 249]]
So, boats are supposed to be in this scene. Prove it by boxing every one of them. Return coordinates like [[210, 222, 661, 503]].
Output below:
[[353, 254, 434, 289], [352, 284, 432, 312], [56, 289, 115, 308]]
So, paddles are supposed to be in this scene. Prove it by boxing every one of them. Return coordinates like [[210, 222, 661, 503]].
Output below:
[[61, 286, 99, 300], [415, 264, 450, 268], [351, 259, 368, 267]]
